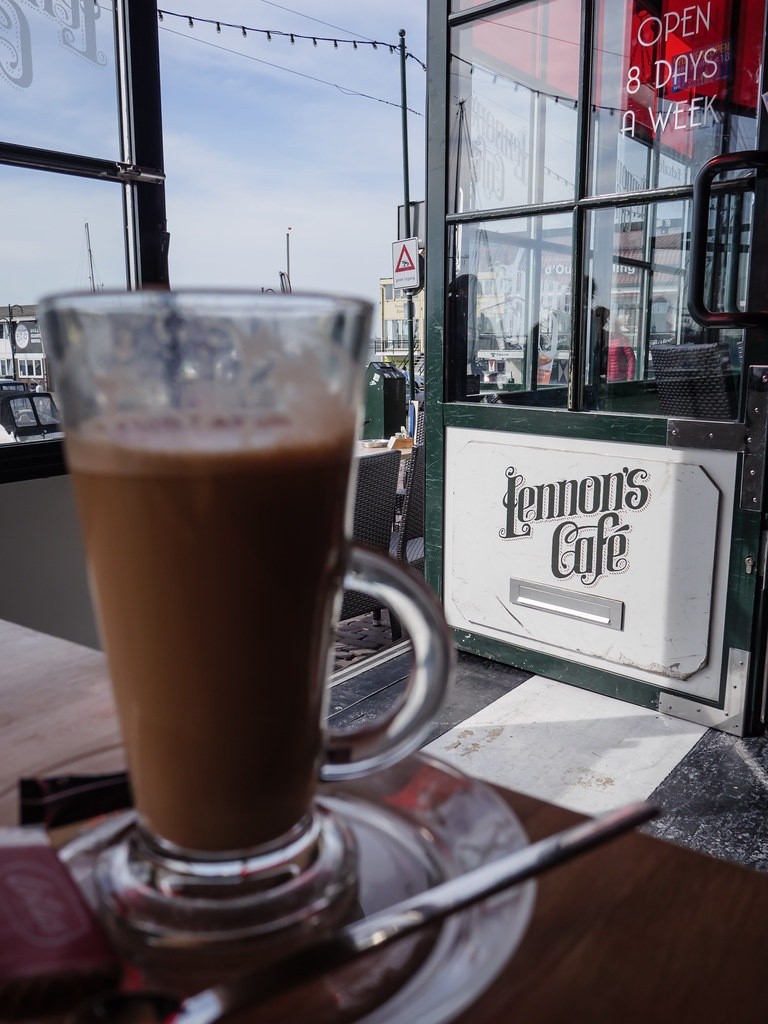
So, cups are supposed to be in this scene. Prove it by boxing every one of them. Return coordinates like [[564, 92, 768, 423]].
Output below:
[[35, 285, 453, 887]]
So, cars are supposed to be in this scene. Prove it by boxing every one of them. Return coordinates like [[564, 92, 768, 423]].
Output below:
[[403, 373, 425, 412]]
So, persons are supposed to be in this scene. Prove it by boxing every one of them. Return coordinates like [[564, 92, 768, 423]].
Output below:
[[455, 274, 505, 381], [521, 272, 608, 391], [592, 307, 612, 376], [605, 312, 636, 382]]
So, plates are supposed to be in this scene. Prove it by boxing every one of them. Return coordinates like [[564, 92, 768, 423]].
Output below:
[[54, 751, 536, 1024]]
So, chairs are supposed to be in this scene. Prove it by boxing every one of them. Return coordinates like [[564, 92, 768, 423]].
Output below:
[[327, 412, 426, 644], [650, 342, 732, 419]]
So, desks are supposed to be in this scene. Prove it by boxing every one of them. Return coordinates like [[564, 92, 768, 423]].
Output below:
[[0, 617, 768, 1023], [358, 438, 413, 459]]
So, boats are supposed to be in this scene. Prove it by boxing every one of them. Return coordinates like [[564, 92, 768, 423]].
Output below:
[[0, 379, 66, 444]]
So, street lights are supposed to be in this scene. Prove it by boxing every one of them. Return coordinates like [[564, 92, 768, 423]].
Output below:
[[0, 304, 38, 379]]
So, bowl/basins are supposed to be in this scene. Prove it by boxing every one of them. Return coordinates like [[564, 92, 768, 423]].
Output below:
[[362, 438, 388, 448]]
